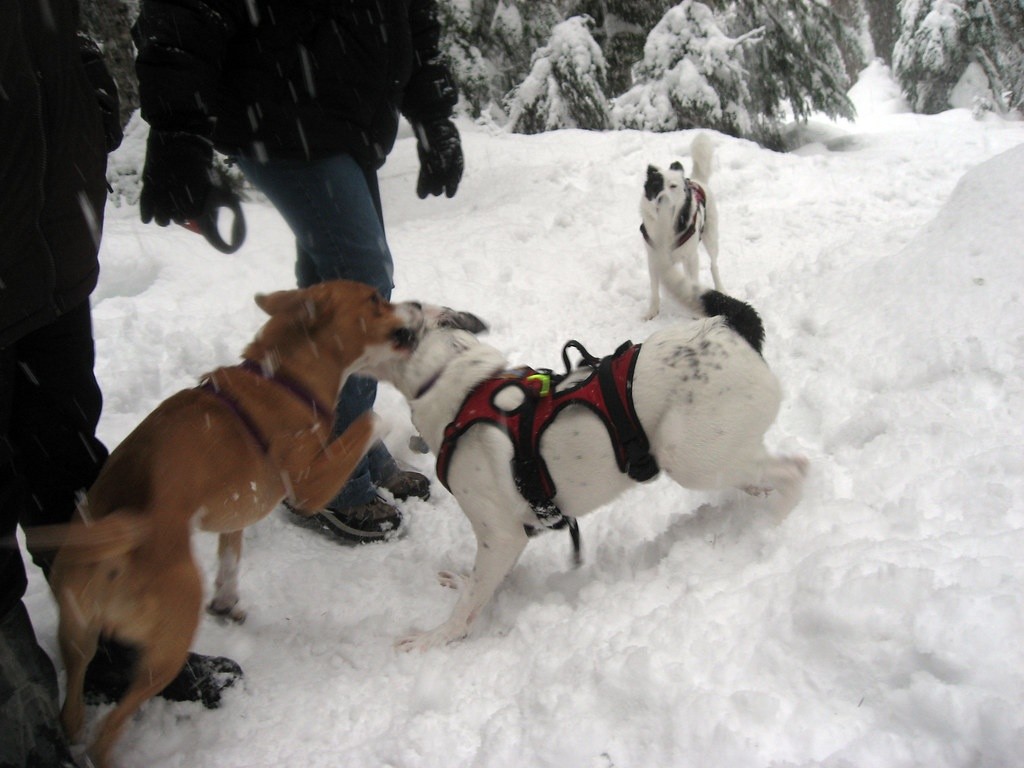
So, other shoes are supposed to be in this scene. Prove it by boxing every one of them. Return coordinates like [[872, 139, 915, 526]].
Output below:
[[87, 643, 242, 710], [0, 643, 67, 767]]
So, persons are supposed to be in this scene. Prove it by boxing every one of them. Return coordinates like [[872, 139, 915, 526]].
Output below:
[[0, 0, 243, 768], [129, 0, 464, 546]]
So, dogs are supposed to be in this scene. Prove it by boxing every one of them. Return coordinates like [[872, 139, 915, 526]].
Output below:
[[21, 130, 811, 768]]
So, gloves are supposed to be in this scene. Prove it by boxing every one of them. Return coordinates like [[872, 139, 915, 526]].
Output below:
[[411, 114, 467, 199], [139, 129, 220, 226]]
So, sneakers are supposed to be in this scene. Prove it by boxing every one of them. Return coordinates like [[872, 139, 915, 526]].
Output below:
[[373, 455, 432, 502], [283, 490, 404, 542]]
